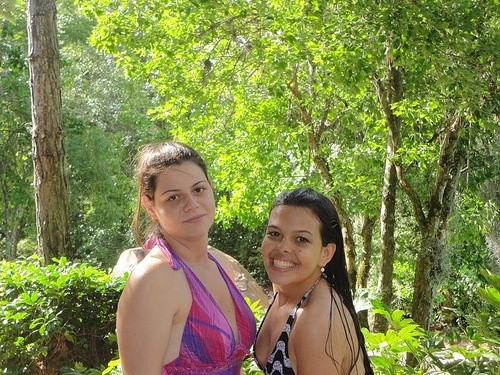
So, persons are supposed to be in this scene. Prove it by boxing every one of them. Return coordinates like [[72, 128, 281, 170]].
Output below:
[[116, 140, 270, 375], [251, 187, 376, 375]]
